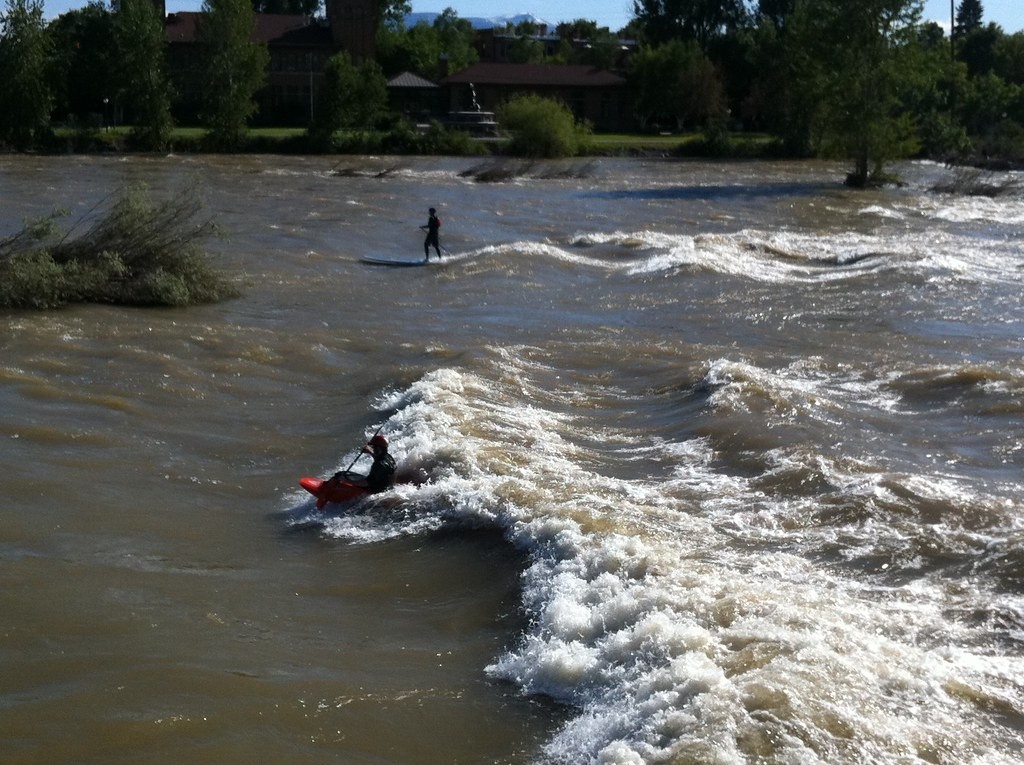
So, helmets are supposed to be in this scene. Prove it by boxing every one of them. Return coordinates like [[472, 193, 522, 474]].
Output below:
[[367, 435, 390, 448]]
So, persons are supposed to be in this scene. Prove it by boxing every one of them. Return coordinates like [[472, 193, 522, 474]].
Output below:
[[422, 208, 441, 261], [353, 435, 397, 493]]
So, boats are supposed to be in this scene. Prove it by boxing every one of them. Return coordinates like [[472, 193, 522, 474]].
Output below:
[[299, 471, 371, 506]]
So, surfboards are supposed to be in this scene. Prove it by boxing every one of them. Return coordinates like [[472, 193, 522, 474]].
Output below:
[[359, 255, 426, 266]]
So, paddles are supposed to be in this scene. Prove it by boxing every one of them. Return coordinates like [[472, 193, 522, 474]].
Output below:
[[420, 227, 450, 254], [317, 421, 389, 508]]
